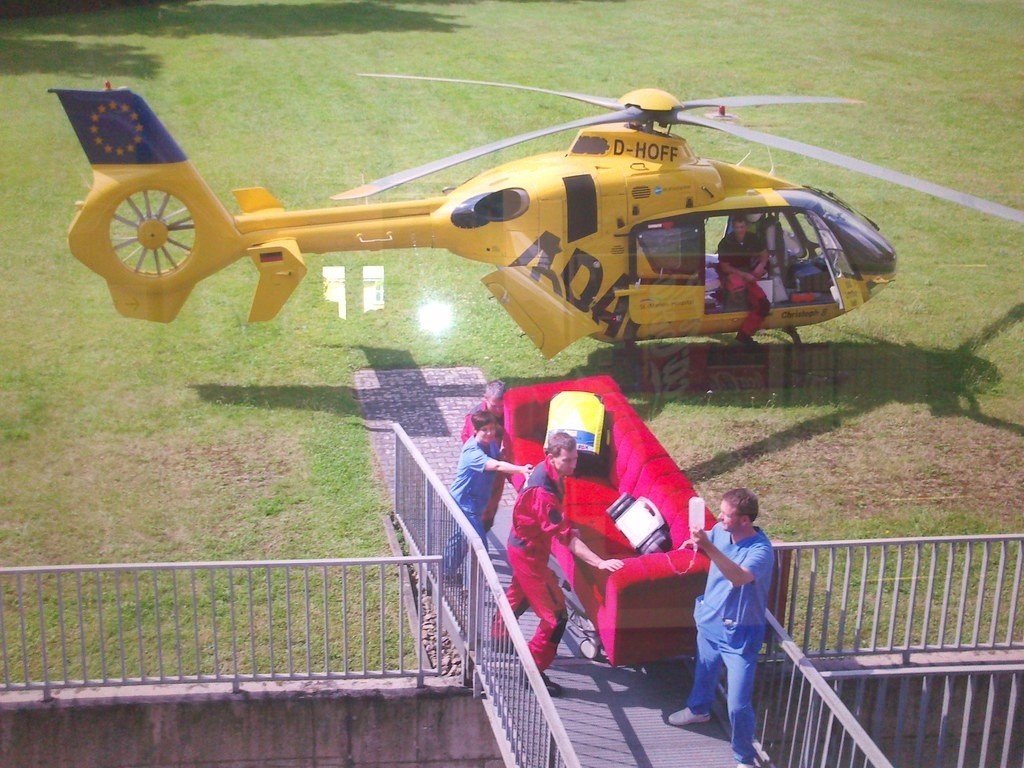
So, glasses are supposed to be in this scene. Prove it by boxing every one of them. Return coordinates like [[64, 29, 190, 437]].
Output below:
[[488, 400, 505, 410], [478, 427, 496, 431]]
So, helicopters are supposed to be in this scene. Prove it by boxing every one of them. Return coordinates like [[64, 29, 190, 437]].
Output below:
[[44, 70, 1023, 392]]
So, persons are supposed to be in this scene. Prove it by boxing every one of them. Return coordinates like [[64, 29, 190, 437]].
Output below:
[[491, 433, 624, 696], [714, 215, 769, 345], [668, 488, 774, 768], [461, 380, 505, 446], [441, 409, 533, 606]]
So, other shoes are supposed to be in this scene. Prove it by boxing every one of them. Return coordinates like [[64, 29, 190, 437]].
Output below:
[[735, 330, 767, 347], [443, 573, 466, 587], [668, 706, 712, 726], [737, 762, 755, 768], [490, 639, 517, 653], [524, 675, 564, 696]]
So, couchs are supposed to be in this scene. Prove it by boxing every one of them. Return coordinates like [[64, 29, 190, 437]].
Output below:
[[502, 375, 720, 667]]
